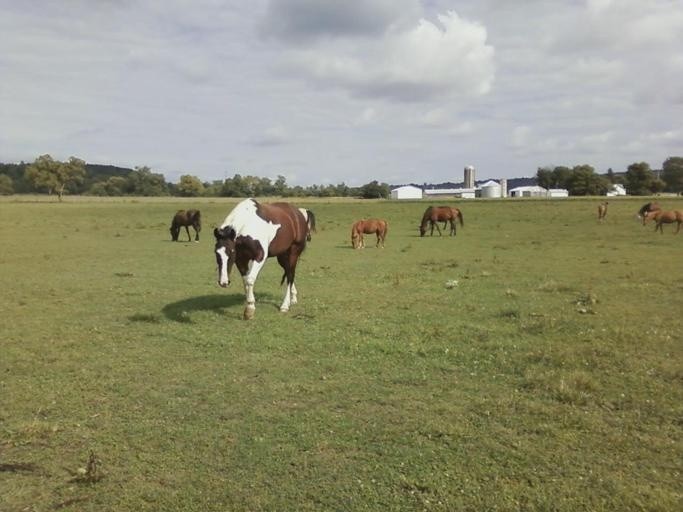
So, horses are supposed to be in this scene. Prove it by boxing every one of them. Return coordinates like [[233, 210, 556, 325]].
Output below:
[[169, 209, 202, 242], [637, 201, 683, 235], [598, 201, 609, 222], [418, 205, 464, 237], [213, 196, 317, 320], [351, 219, 388, 250]]
